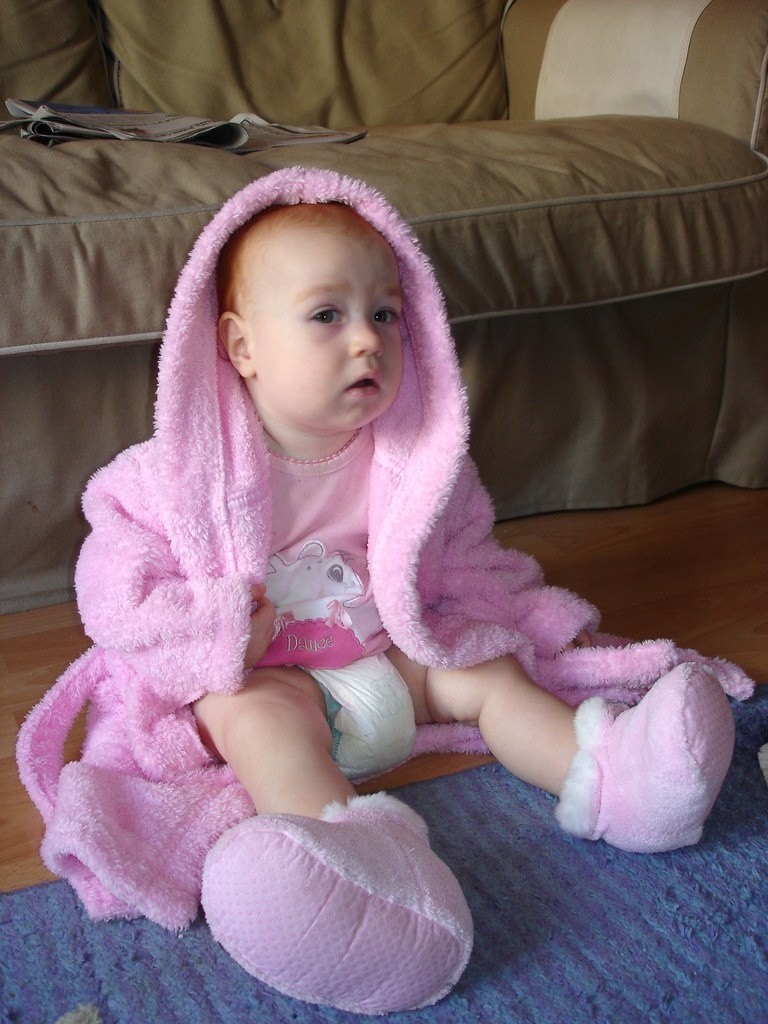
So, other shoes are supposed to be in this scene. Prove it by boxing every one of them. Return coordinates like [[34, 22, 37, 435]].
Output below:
[[201, 791, 474, 1015], [554, 661, 736, 853]]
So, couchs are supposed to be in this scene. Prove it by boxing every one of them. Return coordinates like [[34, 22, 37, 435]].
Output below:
[[0, 0, 768, 615]]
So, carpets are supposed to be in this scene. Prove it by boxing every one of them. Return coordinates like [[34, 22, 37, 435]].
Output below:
[[1, 684, 767, 1023]]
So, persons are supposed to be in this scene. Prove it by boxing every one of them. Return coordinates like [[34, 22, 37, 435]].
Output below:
[[15, 165, 753, 1015]]
